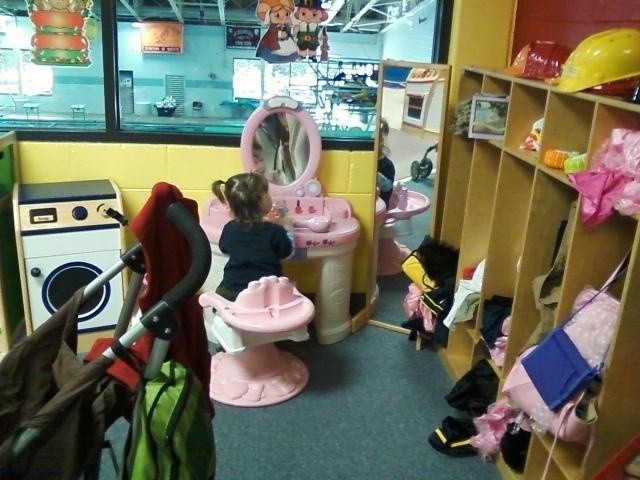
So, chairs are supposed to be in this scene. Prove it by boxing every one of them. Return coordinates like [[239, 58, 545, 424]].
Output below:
[[198, 276, 315, 409]]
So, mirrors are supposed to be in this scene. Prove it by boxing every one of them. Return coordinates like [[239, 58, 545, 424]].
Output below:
[[366, 60, 451, 335], [240, 94, 321, 197]]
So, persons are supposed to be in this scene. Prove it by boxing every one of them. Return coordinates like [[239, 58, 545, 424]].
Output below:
[[212, 173, 294, 302], [377, 119, 394, 212], [256, 1, 330, 63]]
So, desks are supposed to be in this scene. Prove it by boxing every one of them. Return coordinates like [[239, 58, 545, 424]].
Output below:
[[70, 104, 86, 120], [23, 102, 40, 121], [199, 194, 362, 349]]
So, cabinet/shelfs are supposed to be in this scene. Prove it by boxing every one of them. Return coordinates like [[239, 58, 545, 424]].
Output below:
[[432, 68, 640, 480]]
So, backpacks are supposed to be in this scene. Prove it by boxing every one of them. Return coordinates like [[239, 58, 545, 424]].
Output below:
[[110, 339, 216, 479]]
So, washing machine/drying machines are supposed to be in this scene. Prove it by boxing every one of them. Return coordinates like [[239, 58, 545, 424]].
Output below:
[[10, 177, 124, 360]]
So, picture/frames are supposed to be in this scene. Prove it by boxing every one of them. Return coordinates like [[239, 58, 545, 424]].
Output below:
[[468, 94, 509, 139]]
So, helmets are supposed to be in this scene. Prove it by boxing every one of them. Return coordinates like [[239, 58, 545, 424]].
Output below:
[[502, 27, 640, 95]]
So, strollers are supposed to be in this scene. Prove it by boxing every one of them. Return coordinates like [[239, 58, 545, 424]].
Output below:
[[1, 193, 213, 480]]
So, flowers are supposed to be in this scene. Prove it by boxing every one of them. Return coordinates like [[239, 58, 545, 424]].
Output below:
[[155, 96, 177, 108]]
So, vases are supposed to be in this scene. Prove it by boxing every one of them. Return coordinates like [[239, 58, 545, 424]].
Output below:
[[157, 107, 175, 117]]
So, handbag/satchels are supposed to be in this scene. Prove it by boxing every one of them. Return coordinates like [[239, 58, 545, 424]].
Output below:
[[500, 284, 623, 443]]
